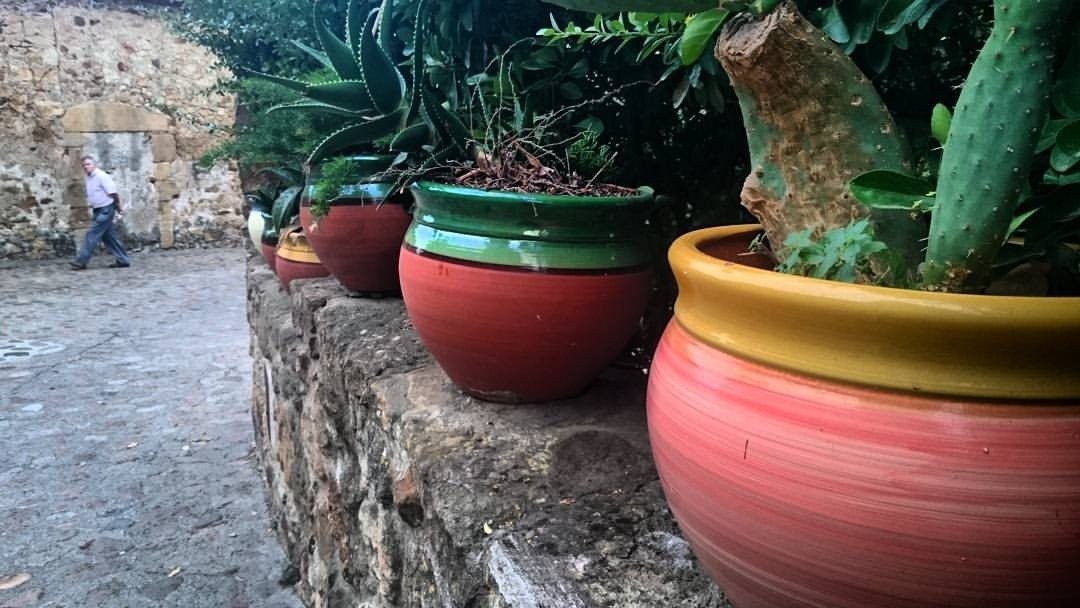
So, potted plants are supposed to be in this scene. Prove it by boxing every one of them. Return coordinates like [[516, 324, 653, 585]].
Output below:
[[193, 0, 1080, 608]]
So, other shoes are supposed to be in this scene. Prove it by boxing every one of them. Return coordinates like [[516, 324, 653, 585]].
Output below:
[[109, 260, 130, 268], [69, 260, 86, 270]]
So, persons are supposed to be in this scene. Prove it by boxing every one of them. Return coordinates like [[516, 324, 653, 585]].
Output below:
[[70, 155, 130, 268]]
